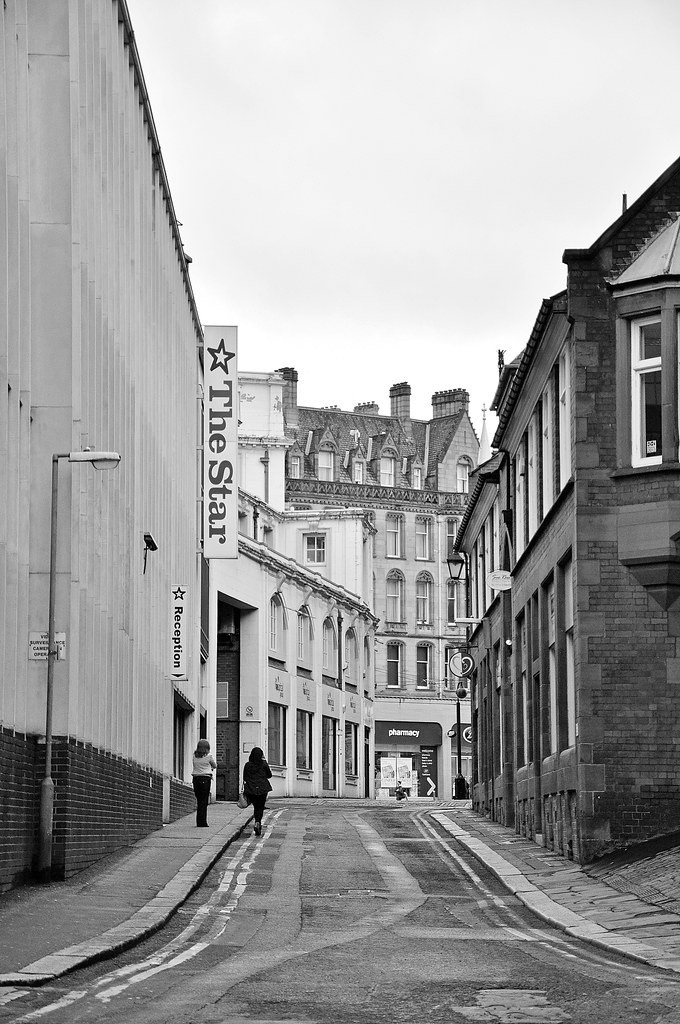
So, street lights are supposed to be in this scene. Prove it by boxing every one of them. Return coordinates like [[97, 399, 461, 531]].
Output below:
[[455, 681, 471, 800], [36, 450, 120, 882]]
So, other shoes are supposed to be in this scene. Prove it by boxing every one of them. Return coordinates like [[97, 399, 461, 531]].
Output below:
[[254, 822, 261, 836]]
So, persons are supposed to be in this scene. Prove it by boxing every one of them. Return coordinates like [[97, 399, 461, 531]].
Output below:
[[191, 739, 217, 827], [394, 780, 409, 801], [243, 746, 273, 835]]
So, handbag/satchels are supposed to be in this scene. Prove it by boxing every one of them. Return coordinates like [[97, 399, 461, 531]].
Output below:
[[237, 780, 251, 808]]
